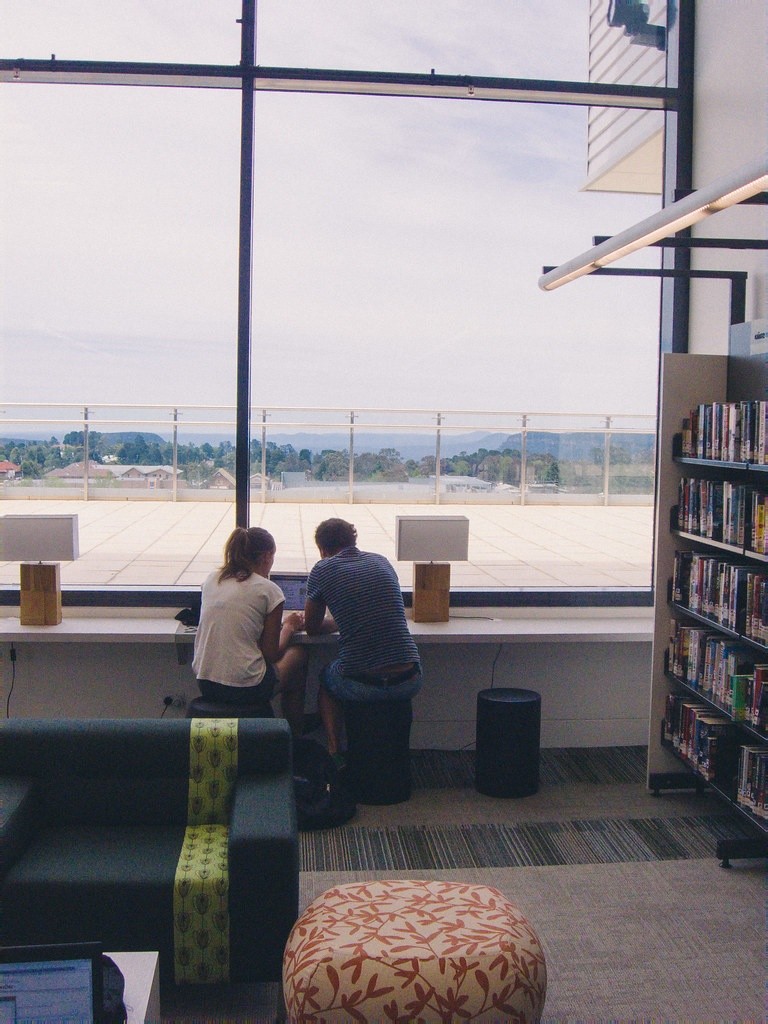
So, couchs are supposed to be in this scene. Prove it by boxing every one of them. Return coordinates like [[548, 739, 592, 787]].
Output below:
[[0, 717, 299, 987]]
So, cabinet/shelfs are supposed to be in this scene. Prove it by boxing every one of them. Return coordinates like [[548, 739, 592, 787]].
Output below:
[[646, 318, 768, 868]]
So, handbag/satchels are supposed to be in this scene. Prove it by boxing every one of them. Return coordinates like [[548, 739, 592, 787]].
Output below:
[[294, 740, 355, 829]]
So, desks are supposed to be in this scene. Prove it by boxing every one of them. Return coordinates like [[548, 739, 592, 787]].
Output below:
[[100, 951, 161, 1024]]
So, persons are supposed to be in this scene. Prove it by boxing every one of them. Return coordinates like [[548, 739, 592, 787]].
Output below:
[[304, 518, 423, 772], [191, 526, 309, 732]]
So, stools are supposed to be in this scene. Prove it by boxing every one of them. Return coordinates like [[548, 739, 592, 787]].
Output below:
[[186, 697, 275, 718], [282, 879, 548, 1024], [345, 700, 413, 805], [476, 688, 541, 798]]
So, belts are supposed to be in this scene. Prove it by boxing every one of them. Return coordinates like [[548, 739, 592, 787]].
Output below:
[[344, 664, 418, 686]]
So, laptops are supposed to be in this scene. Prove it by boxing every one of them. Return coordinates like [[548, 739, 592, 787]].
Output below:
[[0, 942, 106, 1024], [267, 571, 310, 630]]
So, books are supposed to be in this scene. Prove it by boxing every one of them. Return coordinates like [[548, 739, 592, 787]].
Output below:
[[663, 616, 768, 823], [671, 550, 768, 645], [681, 398, 768, 465], [677, 474, 768, 557]]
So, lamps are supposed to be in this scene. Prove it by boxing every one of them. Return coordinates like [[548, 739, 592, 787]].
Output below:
[[396, 516, 469, 622], [0, 514, 79, 625]]
